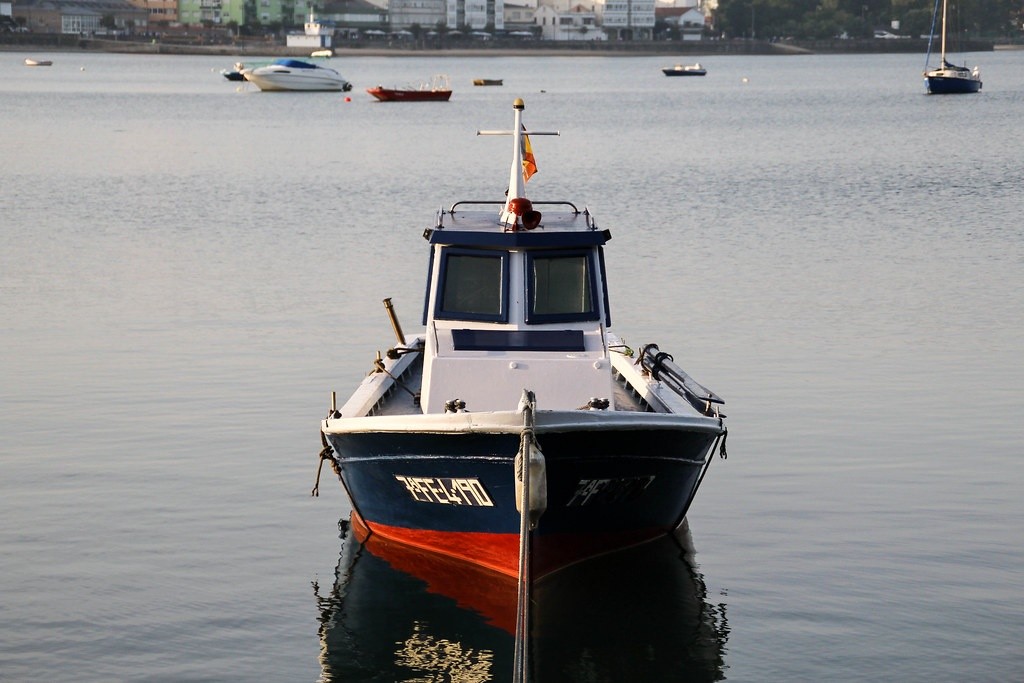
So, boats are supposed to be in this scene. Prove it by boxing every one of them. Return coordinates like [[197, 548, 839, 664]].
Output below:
[[224, 70, 247, 81], [473, 78, 505, 86], [368, 86, 451, 101], [663, 65, 707, 77], [242, 59, 353, 92], [311, 52, 332, 58], [27, 60, 52, 65], [321, 98, 723, 586], [317, 508, 727, 682]]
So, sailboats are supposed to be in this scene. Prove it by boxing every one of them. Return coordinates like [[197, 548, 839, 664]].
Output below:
[[922, 0, 984, 95]]
